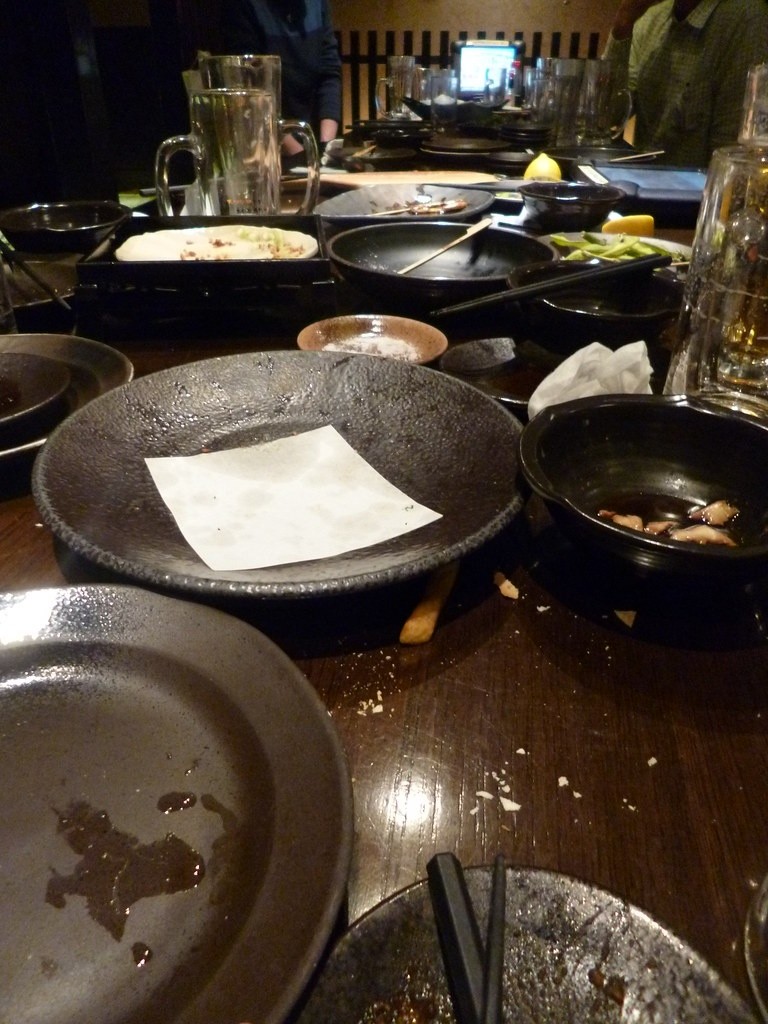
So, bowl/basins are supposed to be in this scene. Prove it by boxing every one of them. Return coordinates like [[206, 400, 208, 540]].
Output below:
[[517, 182, 626, 233], [0, 202, 131, 256], [326, 221, 559, 309], [542, 146, 657, 186], [518, 390, 768, 602], [328, 147, 417, 173], [505, 260, 683, 358]]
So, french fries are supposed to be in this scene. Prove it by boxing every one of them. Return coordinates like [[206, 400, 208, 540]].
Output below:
[[398, 560, 457, 644]]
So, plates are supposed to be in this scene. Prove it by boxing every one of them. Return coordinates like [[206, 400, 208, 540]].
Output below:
[[744, 874, 768, 1024], [297, 313, 448, 364], [288, 865, 755, 1023], [440, 336, 577, 416], [0, 586, 357, 1024], [537, 232, 693, 281], [419, 137, 511, 166], [0, 333, 135, 458], [116, 227, 318, 259], [1, 260, 77, 312], [312, 183, 494, 220], [31, 350, 525, 601]]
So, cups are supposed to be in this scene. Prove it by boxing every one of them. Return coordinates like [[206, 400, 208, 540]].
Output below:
[[155, 87, 279, 216], [199, 54, 319, 216], [373, 54, 633, 147]]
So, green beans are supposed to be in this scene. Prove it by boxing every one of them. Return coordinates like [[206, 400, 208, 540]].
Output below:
[[548, 230, 684, 264]]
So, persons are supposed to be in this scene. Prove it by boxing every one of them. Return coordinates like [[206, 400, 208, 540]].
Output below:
[[151, 0, 344, 212], [570, 2, 768, 166]]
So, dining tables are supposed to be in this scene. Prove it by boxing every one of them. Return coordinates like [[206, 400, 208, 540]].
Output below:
[[0, 188, 768, 1013]]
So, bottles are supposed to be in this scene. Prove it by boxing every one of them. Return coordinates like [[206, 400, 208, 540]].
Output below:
[[660, 60, 767, 422]]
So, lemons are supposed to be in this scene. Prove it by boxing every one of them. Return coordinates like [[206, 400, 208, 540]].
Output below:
[[601, 214, 654, 238], [523, 153, 562, 180]]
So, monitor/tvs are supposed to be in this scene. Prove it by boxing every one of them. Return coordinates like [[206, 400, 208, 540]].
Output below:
[[449, 40, 526, 102]]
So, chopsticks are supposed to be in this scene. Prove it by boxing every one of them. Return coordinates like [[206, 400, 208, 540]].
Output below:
[[352, 145, 377, 159], [426, 851, 506, 1024], [429, 251, 672, 315]]
